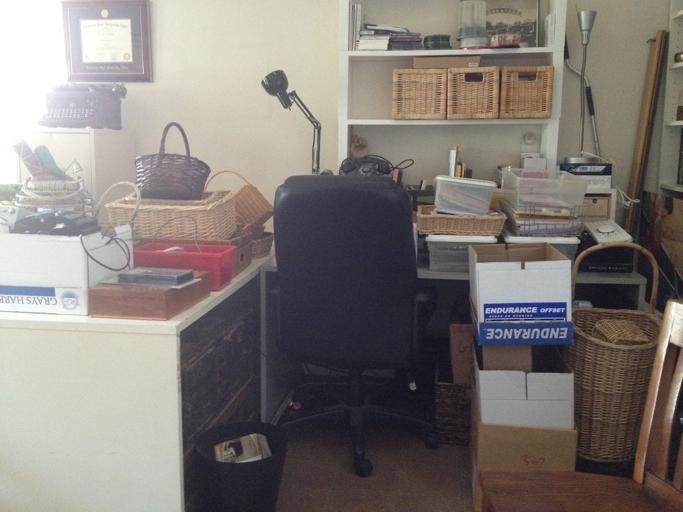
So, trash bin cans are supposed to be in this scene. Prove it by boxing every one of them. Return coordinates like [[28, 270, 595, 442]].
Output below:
[[193, 422, 288, 512]]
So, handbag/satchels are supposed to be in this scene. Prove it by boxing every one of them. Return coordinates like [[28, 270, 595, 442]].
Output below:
[[202, 169, 273, 237]]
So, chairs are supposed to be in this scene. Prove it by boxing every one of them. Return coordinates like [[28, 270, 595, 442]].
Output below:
[[273, 174, 439, 477], [478, 298, 683, 510]]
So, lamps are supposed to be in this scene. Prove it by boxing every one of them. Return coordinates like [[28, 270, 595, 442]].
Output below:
[[261, 69, 321, 174], [577, 10, 596, 158]]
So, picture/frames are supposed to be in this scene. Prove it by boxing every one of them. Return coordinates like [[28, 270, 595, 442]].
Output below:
[[62, 1, 154, 84]]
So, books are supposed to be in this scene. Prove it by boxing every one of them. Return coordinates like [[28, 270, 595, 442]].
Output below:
[[350, 2, 425, 50]]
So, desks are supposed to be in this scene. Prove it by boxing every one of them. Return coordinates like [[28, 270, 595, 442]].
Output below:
[[419, 271, 648, 325]]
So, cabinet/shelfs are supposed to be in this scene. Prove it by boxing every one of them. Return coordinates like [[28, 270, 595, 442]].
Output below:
[[1, 250, 296, 511], [338, 0, 568, 176]]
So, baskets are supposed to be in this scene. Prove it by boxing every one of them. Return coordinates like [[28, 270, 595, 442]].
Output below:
[[499, 64, 555, 120], [414, 204, 507, 236], [237, 230, 274, 260], [13, 175, 95, 218], [103, 187, 238, 243], [568, 237, 663, 465], [390, 67, 447, 121], [183, 234, 254, 280], [125, 121, 212, 201], [446, 65, 499, 120], [589, 317, 654, 349]]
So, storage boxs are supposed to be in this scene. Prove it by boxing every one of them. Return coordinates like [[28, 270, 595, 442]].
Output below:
[[446, 242, 579, 512], [426, 234, 498, 273], [1, 224, 134, 316], [502, 224, 581, 272], [500, 167, 588, 212], [435, 174, 498, 218], [234, 243, 251, 276], [134, 243, 236, 291]]
